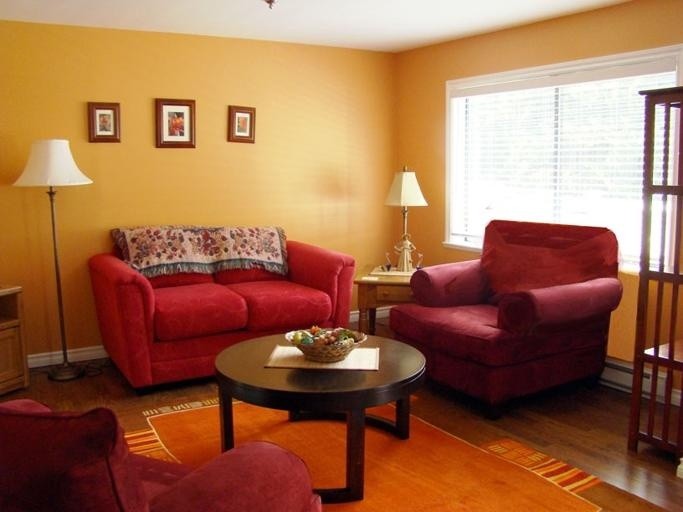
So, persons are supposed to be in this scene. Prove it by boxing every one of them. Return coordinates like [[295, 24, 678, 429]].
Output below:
[[394, 233, 417, 272]]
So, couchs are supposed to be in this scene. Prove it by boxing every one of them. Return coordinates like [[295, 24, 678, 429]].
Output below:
[[87, 226, 357, 392]]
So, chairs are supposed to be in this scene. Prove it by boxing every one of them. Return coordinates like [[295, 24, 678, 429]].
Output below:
[[0, 398, 323, 511], [388, 218, 623, 420]]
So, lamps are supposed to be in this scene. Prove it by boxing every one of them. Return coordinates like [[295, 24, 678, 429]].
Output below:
[[385, 163, 429, 272], [12, 138, 95, 382]]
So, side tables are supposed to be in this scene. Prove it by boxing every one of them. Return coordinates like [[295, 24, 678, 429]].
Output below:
[[353, 274, 414, 335]]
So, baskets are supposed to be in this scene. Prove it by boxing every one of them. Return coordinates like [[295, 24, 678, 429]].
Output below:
[[284, 328, 367, 363]]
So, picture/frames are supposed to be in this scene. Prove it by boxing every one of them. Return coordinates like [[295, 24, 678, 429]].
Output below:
[[228, 105, 256, 143], [87, 102, 120, 142], [156, 98, 195, 148]]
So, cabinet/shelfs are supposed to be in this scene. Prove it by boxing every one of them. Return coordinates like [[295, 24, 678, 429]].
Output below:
[[0, 284, 31, 395]]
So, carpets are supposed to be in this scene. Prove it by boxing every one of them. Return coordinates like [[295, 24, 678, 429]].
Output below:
[[121, 395, 670, 512]]
